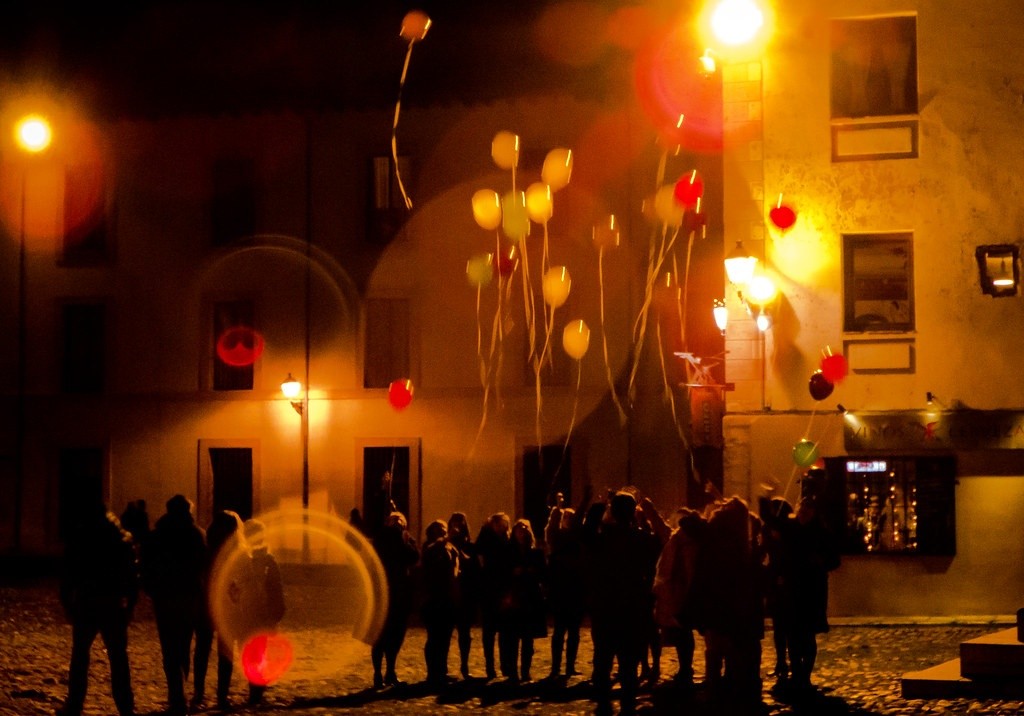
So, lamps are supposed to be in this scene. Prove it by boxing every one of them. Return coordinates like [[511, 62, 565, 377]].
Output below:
[[837, 404, 848, 415], [281, 373, 304, 415], [992, 257, 1014, 286], [727, 240, 777, 330], [926, 392, 935, 405]]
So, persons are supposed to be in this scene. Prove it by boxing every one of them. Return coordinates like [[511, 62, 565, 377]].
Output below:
[[55, 494, 285, 716], [354, 487, 842, 716], [848, 491, 908, 550]]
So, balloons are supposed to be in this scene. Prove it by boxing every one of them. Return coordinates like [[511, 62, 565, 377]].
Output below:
[[594, 216, 620, 250], [563, 319, 590, 361], [401, 13, 429, 40], [771, 204, 796, 230], [792, 439, 824, 479], [810, 353, 848, 400], [643, 169, 703, 229], [465, 130, 574, 311], [388, 378, 414, 410]]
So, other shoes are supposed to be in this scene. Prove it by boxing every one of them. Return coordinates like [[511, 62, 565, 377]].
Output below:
[[373, 661, 815, 716], [218, 699, 237, 712], [190, 697, 207, 709], [118, 709, 138, 716], [155, 707, 187, 716], [57, 705, 81, 716]]
